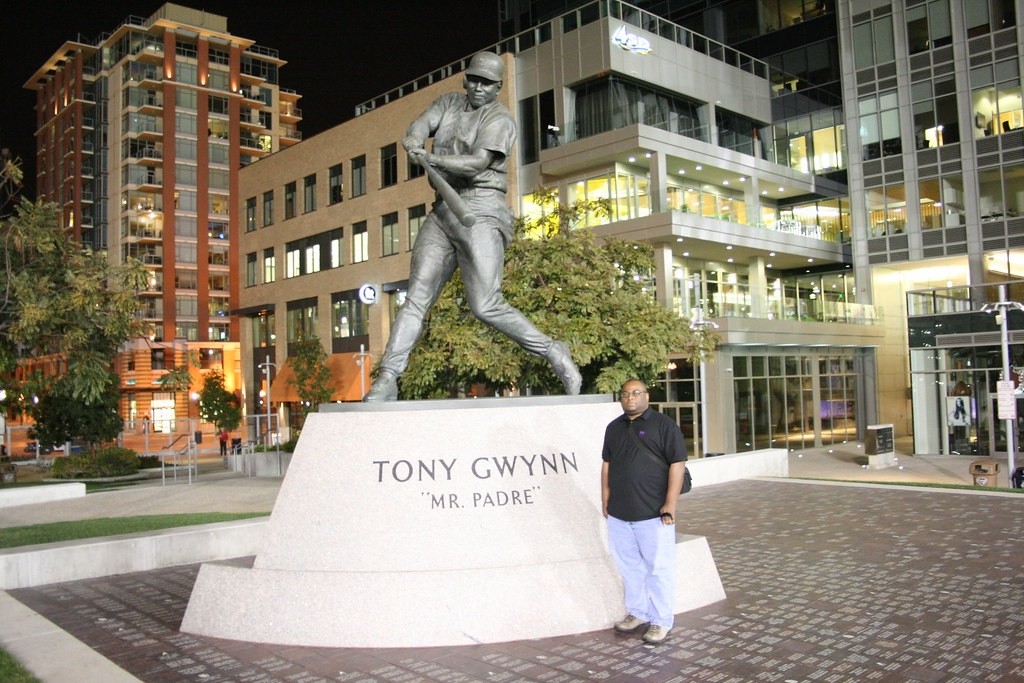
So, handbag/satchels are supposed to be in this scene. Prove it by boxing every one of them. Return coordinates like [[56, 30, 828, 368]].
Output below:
[[677, 466, 693, 494]]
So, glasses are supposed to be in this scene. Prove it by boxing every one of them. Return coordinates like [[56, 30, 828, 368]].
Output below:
[[620, 390, 647, 398]]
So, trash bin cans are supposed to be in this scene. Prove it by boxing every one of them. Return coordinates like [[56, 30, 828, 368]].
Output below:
[[969, 460, 1001, 488], [231, 438, 241, 454], [864, 423, 896, 456], [0, 462, 18, 483]]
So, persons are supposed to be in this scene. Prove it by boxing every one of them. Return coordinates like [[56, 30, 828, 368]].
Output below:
[[363, 50, 583, 401], [602, 379, 688, 643], [218, 428, 228, 455]]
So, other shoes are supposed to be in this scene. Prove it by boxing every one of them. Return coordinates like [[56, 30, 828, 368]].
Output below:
[[614, 615, 650, 633], [643, 622, 672, 643]]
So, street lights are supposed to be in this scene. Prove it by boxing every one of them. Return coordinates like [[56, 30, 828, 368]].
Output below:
[[353, 344, 373, 400], [258, 355, 278, 449]]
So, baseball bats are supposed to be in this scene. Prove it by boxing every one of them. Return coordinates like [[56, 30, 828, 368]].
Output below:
[[416, 153, 476, 227]]
